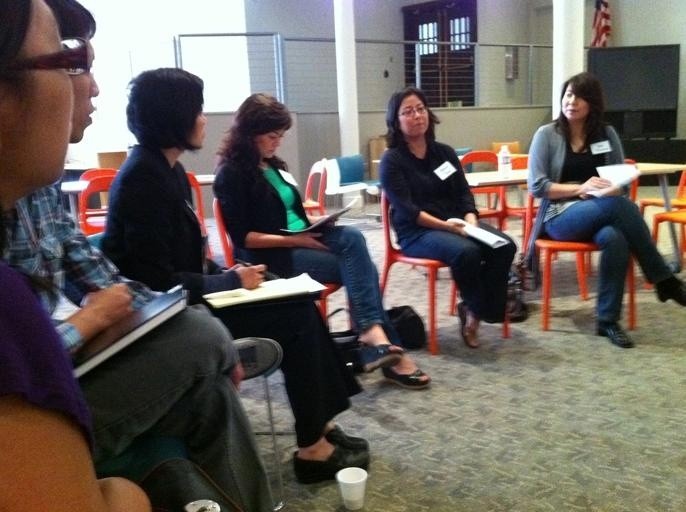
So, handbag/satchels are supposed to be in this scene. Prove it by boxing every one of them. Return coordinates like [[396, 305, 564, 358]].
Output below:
[[326, 328, 360, 367], [504, 272, 532, 324], [382, 304, 430, 351], [132, 454, 248, 512]]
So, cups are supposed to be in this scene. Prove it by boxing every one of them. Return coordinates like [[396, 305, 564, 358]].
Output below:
[[335, 467, 368, 511]]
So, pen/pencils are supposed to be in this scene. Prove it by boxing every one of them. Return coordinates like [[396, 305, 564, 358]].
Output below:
[[234, 258, 280, 279], [79, 277, 104, 292]]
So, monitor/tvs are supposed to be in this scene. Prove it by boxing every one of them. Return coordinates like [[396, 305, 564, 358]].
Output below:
[[587, 43, 681, 114]]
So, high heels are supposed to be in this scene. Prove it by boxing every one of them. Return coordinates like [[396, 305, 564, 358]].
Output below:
[[354, 336, 405, 372], [382, 365, 434, 390]]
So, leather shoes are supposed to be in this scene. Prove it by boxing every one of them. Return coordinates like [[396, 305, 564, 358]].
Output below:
[[321, 425, 370, 453], [289, 442, 373, 485], [591, 313, 638, 351], [650, 275, 685, 309]]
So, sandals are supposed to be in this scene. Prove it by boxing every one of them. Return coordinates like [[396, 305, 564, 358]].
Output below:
[[454, 301, 485, 352]]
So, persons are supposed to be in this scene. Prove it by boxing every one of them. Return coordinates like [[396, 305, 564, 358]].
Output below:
[[520, 72, 686, 347], [2, 2, 152, 512], [378, 86, 517, 347], [9, 0, 281, 510], [213, 92, 430, 390], [98, 68, 369, 485]]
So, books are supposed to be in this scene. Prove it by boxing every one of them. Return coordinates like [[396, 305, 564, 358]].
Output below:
[[67, 285, 190, 379]]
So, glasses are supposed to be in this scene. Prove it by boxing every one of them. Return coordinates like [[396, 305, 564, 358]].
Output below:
[[396, 105, 428, 118], [4, 37, 93, 77]]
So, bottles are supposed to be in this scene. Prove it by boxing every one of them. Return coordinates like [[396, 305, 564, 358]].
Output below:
[[497, 145, 513, 178]]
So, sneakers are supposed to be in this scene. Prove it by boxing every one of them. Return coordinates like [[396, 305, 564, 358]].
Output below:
[[229, 330, 285, 383]]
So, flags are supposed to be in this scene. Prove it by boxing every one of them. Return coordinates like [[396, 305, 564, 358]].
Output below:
[[590, 2, 612, 47]]
